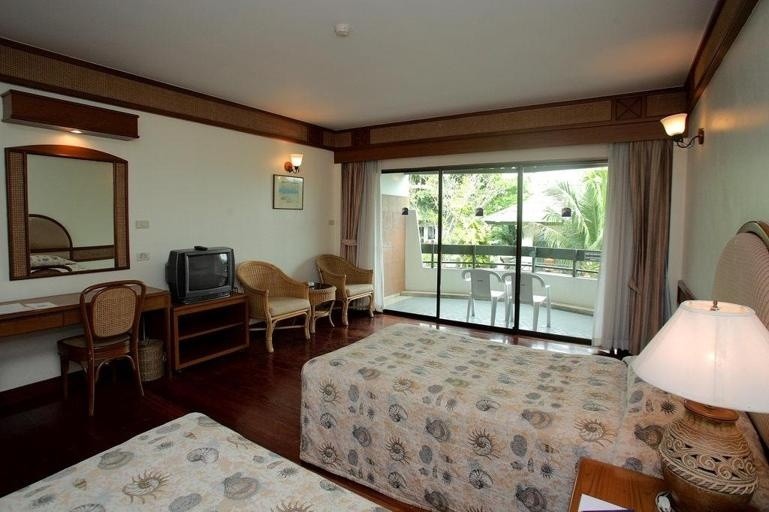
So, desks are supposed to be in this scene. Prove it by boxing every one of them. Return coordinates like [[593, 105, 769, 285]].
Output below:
[[0, 282, 173, 385]]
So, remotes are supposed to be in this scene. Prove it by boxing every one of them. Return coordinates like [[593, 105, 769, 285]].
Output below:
[[194, 245, 209, 252]]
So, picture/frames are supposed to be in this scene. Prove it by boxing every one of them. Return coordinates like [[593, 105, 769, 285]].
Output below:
[[271, 173, 305, 210]]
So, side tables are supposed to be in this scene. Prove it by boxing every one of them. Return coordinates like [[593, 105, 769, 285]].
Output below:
[[308, 281, 337, 334]]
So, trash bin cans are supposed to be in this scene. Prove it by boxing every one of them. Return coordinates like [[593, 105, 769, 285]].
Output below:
[[137, 334, 164, 381]]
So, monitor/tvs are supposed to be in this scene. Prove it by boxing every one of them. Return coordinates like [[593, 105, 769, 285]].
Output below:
[[165, 247, 235, 307]]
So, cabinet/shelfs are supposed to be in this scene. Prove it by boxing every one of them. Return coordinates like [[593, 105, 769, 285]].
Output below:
[[173, 293, 251, 371]]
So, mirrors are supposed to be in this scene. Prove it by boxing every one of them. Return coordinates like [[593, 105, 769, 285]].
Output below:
[[4, 144, 128, 282]]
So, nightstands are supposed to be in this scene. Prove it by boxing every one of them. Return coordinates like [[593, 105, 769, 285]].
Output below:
[[567, 454, 767, 512]]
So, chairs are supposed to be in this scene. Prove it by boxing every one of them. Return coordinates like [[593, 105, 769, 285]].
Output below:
[[501, 271, 551, 330], [461, 267, 513, 326], [235, 260, 311, 352], [316, 254, 376, 326], [56, 279, 147, 417]]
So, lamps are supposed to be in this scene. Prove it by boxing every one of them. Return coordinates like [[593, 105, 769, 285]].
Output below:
[[284, 152, 304, 173], [629, 297, 769, 512], [659, 113, 704, 148]]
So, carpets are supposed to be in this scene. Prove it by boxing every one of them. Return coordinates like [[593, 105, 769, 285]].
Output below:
[[0, 410, 395, 512]]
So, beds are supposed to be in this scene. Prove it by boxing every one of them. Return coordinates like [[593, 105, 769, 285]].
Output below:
[[297, 219, 769, 512]]
